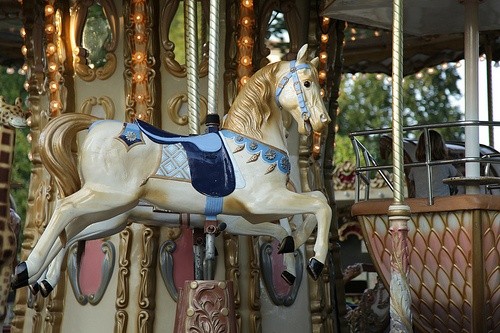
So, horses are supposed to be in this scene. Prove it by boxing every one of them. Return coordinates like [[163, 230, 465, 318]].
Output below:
[[11, 43, 332, 290], [28, 106, 297, 298]]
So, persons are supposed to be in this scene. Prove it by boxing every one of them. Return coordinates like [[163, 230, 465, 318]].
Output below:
[[408, 130, 463, 198]]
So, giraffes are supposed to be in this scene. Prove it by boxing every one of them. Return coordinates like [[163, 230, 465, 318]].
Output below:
[[0, 96, 26, 332]]
